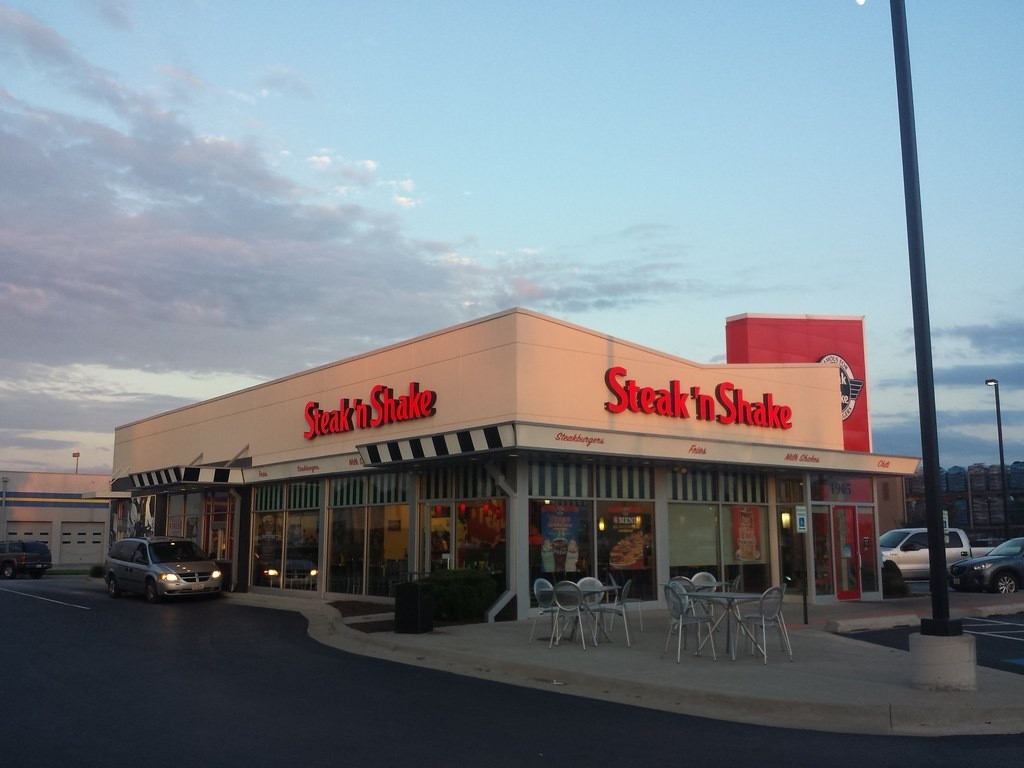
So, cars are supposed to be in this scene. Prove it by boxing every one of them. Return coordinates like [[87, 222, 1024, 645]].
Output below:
[[875, 532, 970, 592], [0, 540, 53, 579], [239, 540, 325, 589], [109, 535, 222, 597], [957, 539, 1023, 600]]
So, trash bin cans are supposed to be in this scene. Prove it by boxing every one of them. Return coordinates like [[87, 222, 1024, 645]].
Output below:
[[394, 571, 435, 636]]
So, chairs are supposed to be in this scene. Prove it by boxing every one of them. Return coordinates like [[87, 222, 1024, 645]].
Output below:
[[340, 559, 408, 597], [528, 571, 793, 665]]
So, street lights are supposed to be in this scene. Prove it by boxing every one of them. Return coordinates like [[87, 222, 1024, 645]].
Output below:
[[982, 378, 1015, 529]]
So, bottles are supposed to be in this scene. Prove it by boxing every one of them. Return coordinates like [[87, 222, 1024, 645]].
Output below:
[[643, 534, 653, 567]]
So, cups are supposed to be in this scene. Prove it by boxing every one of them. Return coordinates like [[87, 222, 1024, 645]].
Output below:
[[552, 537, 568, 571], [540, 551, 556, 572], [739, 511, 756, 560], [565, 552, 580, 571]]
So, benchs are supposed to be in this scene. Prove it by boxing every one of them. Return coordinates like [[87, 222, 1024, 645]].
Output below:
[[272, 559, 316, 585]]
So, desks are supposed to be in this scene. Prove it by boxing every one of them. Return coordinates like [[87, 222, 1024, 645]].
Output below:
[[660, 581, 729, 592], [679, 591, 781, 657], [539, 586, 622, 644]]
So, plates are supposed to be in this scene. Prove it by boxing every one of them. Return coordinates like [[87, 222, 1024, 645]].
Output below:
[[609, 552, 644, 570]]
